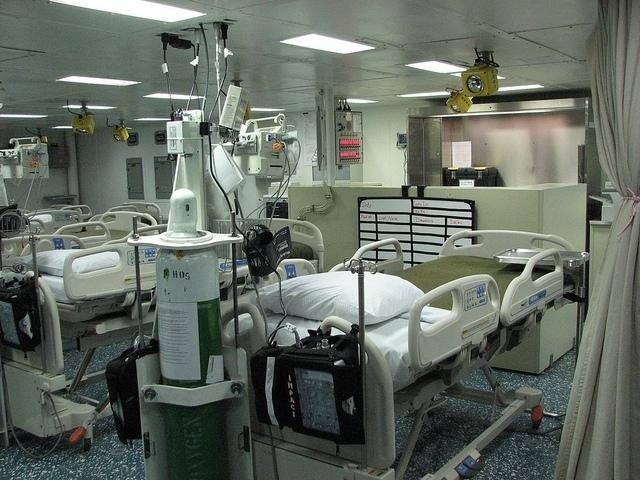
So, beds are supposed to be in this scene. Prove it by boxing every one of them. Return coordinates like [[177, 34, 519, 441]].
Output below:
[[0, 212, 158, 257], [0, 204, 92, 220], [1, 217, 325, 452], [223, 229, 583, 480], [0, 202, 162, 235]]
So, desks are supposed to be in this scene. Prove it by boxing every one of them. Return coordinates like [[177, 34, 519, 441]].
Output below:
[[287, 184, 587, 375]]
